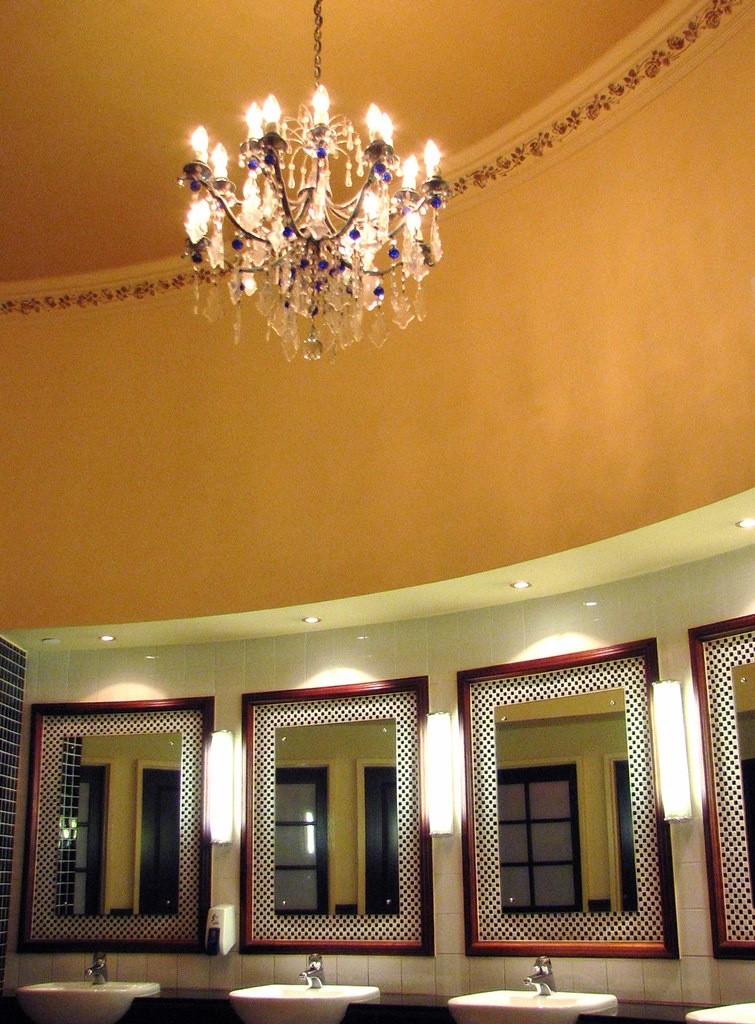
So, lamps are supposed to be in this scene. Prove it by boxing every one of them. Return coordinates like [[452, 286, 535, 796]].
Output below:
[[424, 712, 456, 838], [174, 1, 448, 364], [650, 678, 694, 823], [207, 727, 234, 845]]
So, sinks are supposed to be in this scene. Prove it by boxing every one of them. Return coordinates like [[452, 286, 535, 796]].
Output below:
[[16, 981, 161, 1024], [685, 1003, 755, 1024], [230, 985, 380, 1024], [448, 990, 617, 1024]]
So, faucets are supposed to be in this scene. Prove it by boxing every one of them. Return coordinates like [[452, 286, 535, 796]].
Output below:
[[300, 953, 325, 989], [523, 955, 556, 995], [85, 952, 106, 984]]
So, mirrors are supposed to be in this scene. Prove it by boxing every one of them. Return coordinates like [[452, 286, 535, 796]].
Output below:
[[686, 613, 755, 961], [14, 696, 215, 953], [456, 637, 679, 961], [238, 674, 435, 956]]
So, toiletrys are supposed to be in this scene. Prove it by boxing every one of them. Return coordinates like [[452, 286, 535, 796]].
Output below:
[[204, 904, 236, 959]]
